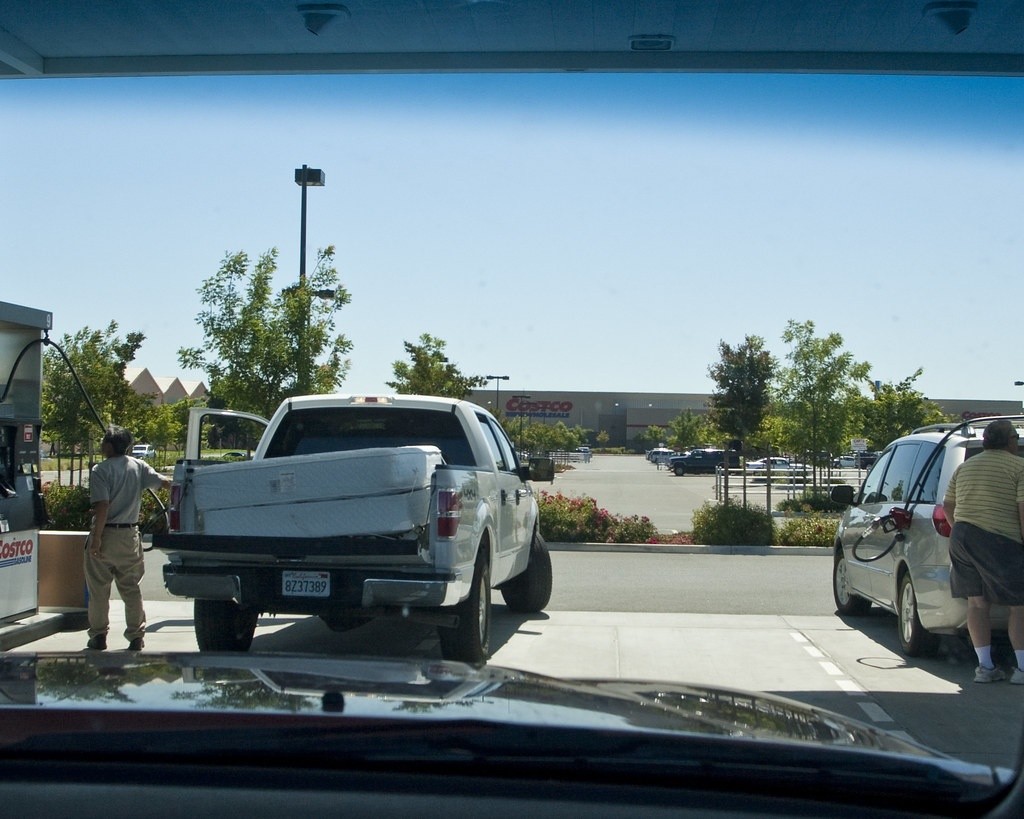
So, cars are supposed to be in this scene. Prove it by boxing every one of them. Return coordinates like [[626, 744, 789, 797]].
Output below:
[[222, 452, 246, 457], [831, 455, 855, 469], [577, 446, 592, 458], [745, 457, 790, 469], [131, 444, 156, 460]]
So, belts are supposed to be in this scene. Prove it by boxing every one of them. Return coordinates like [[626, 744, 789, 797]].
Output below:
[[105, 523, 138, 529]]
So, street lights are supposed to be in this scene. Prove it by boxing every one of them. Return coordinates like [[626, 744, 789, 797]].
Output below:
[[511, 395, 531, 462], [283, 287, 335, 360], [486, 376, 510, 411], [292, 162, 326, 286]]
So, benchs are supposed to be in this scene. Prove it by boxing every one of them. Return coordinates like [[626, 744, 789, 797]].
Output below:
[[295, 434, 475, 466]]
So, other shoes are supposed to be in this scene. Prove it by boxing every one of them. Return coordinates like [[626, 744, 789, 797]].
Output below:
[[130, 638, 144, 651], [87, 634, 107, 650]]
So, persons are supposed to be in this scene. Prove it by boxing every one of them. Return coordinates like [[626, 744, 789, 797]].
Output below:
[[82, 426, 173, 650], [942, 420, 1024, 685]]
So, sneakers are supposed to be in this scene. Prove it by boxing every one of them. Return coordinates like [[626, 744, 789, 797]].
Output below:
[[974, 665, 1006, 684], [1010, 667, 1024, 685]]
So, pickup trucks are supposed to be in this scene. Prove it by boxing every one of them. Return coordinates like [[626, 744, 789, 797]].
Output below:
[[152, 392, 554, 667]]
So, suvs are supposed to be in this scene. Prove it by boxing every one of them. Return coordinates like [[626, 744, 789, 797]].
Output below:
[[648, 449, 676, 463], [854, 451, 884, 470], [832, 414, 1024, 659], [669, 449, 740, 476]]
